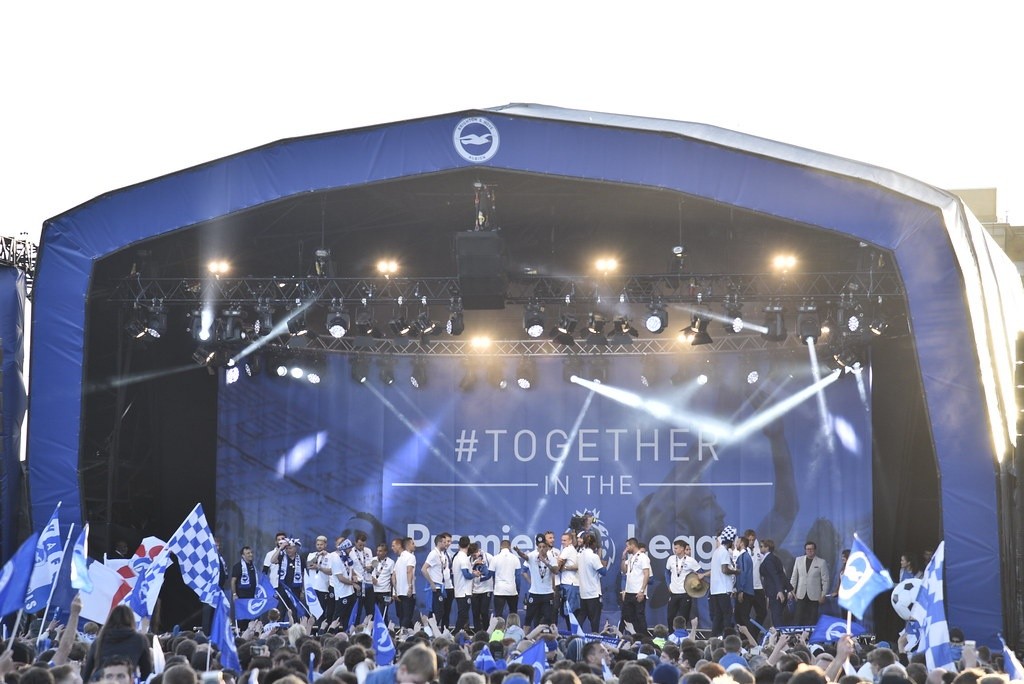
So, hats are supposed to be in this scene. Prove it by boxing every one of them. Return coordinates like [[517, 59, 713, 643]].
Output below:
[[316, 536, 327, 542], [652, 663, 681, 683], [949, 627, 964, 643], [536, 534, 546, 545], [684, 572, 711, 599], [873, 641, 890, 649], [720, 526, 737, 541], [576, 530, 589, 538]]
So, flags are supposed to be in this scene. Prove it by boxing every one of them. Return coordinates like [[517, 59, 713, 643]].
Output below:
[[252, 573, 275, 599], [23, 509, 63, 615], [836, 537, 895, 620], [50, 524, 133, 626], [282, 586, 305, 618], [372, 604, 396, 665], [807, 615, 866, 644], [912, 543, 958, 672], [166, 504, 229, 609], [304, 577, 323, 620], [210, 595, 240, 673], [0, 531, 39, 617], [102, 535, 168, 621], [235, 597, 277, 620]]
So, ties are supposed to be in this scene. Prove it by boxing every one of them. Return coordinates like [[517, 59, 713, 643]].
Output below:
[[445, 551, 452, 568]]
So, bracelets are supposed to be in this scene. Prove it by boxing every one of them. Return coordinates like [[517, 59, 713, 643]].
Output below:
[[640, 590, 644, 592], [565, 566, 567, 570], [559, 570, 562, 573], [622, 559, 625, 560], [233, 593, 236, 596]]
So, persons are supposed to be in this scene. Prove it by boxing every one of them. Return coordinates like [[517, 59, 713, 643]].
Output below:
[[105, 540, 128, 559], [215, 498, 246, 566], [202, 511, 917, 637], [632, 386, 799, 609], [0, 588, 1024, 684], [340, 512, 386, 557]]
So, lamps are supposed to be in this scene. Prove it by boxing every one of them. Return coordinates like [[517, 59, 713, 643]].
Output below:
[[127, 299, 889, 390]]
